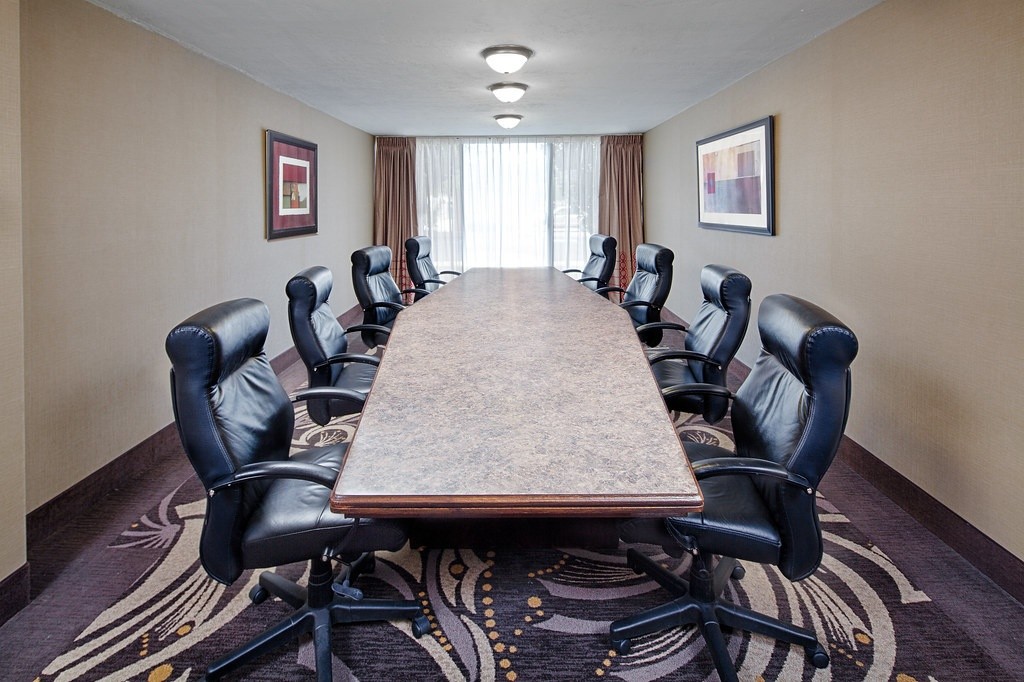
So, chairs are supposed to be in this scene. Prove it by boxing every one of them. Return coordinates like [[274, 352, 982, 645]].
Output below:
[[351, 246, 431, 350], [404, 235, 462, 302], [285, 266, 392, 425], [608, 294, 858, 682], [636, 264, 754, 427], [562, 235, 616, 301], [164, 297, 433, 682], [594, 243, 674, 348]]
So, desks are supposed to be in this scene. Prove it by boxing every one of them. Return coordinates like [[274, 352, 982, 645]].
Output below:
[[329, 266, 704, 550]]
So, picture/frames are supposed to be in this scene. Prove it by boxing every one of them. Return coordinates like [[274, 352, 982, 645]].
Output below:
[[264, 129, 319, 241], [694, 115, 777, 238]]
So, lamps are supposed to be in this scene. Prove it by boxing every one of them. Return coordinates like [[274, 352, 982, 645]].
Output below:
[[493, 113, 524, 130], [480, 44, 535, 76], [486, 84, 530, 104]]
